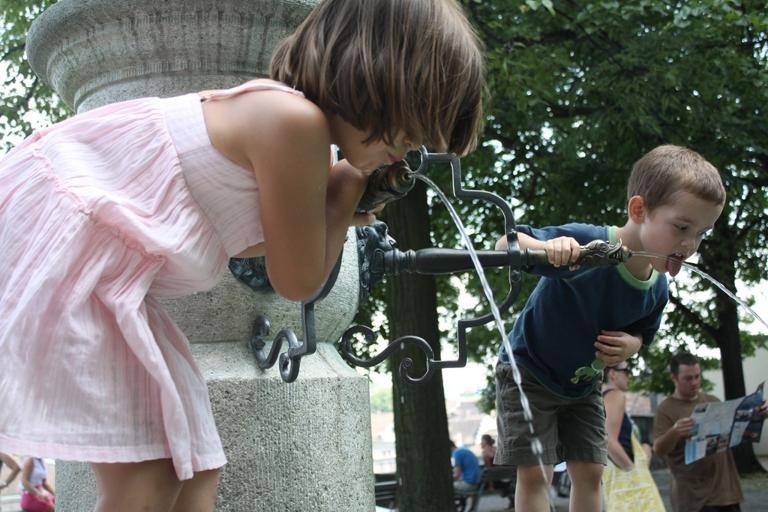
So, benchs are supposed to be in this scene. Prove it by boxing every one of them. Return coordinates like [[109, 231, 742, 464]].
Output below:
[[374, 474, 397, 507], [452, 467, 516, 512]]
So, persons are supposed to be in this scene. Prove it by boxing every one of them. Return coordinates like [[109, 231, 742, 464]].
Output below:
[[449, 440, 480, 490], [0, 0, 483, 512], [0, 450, 55, 512], [492, 144, 727, 512], [481, 435, 496, 490], [599, 359, 666, 512], [653, 353, 768, 512]]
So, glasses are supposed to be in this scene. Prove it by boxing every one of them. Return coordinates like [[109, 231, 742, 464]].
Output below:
[[614, 368, 630, 375]]
